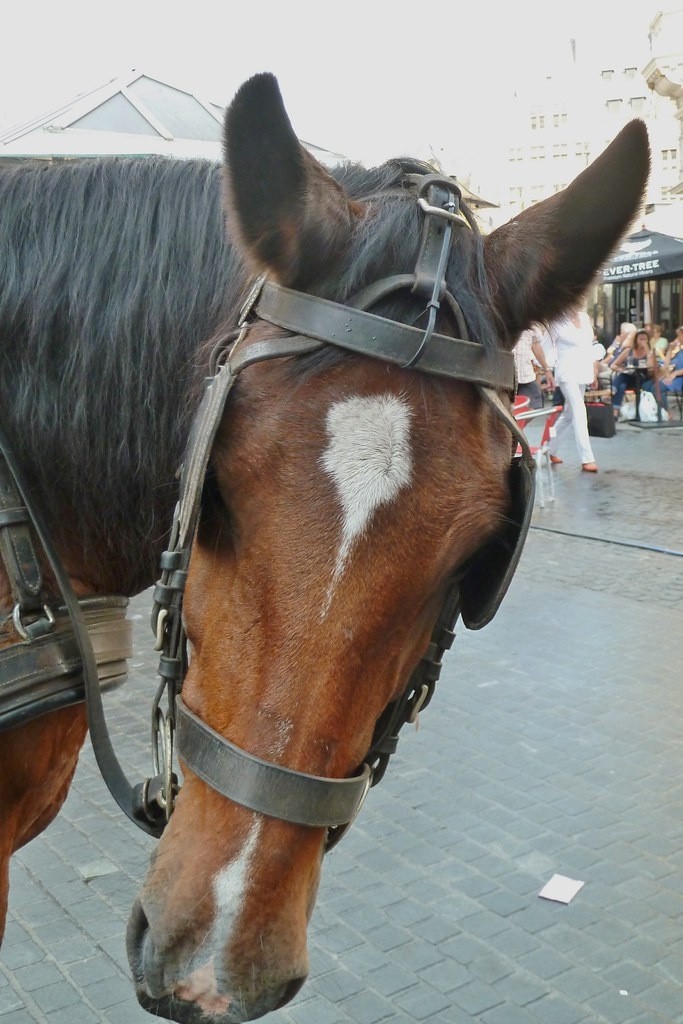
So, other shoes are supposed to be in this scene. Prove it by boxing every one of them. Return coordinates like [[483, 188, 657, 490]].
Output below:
[[550, 455, 563, 463], [582, 463, 598, 471]]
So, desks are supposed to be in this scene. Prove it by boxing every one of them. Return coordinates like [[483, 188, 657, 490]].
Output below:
[[624, 366, 657, 422]]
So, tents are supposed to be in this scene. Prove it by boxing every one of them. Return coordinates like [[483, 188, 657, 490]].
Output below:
[[589, 229, 682, 322]]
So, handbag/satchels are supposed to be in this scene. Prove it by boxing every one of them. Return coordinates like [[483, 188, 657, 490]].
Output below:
[[619, 391, 669, 421], [584, 402, 617, 438]]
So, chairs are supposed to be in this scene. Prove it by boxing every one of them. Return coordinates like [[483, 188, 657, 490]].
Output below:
[[514, 395, 531, 430], [513, 406, 563, 509], [585, 377, 613, 404], [610, 370, 619, 402], [666, 374, 683, 422]]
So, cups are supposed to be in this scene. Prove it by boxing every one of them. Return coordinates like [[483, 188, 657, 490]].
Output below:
[[639, 360, 646, 367], [626, 356, 633, 367]]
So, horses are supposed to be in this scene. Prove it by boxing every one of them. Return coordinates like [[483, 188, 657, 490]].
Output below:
[[0, 72, 654, 1020]]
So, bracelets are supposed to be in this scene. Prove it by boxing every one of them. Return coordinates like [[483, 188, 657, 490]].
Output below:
[[545, 367, 551, 371]]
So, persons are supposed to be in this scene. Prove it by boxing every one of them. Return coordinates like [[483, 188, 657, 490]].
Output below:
[[587, 322, 683, 422], [540, 311, 599, 471], [511, 328, 553, 409]]
